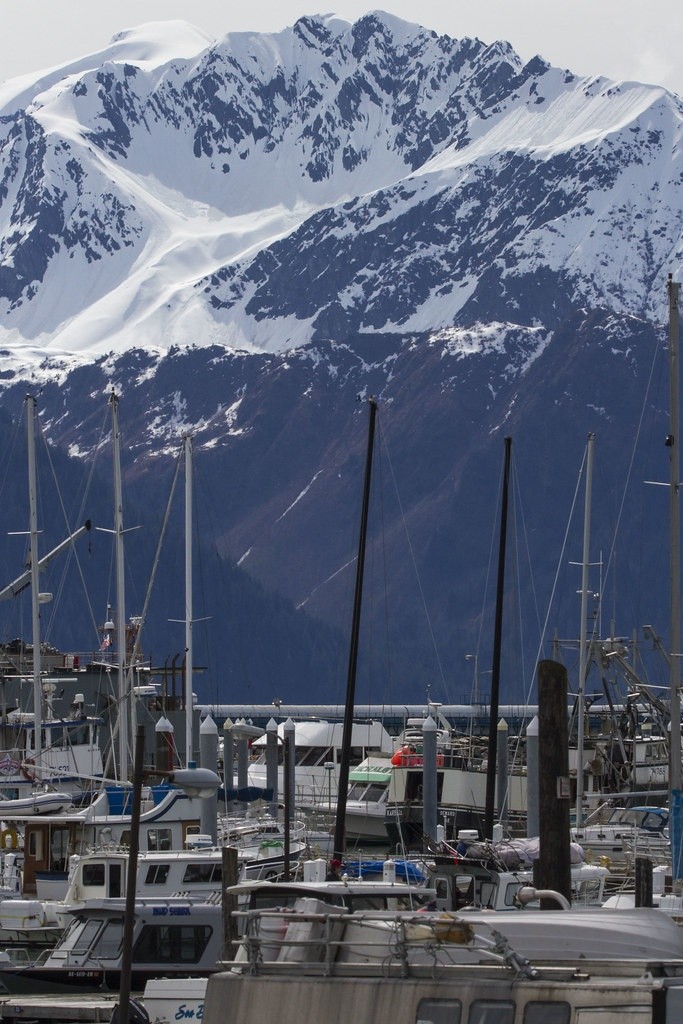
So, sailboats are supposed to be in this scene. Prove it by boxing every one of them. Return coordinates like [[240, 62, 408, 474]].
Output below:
[[0, 271, 683, 1024]]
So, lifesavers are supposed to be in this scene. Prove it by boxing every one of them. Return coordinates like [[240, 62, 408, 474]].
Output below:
[[585, 848, 611, 871]]
